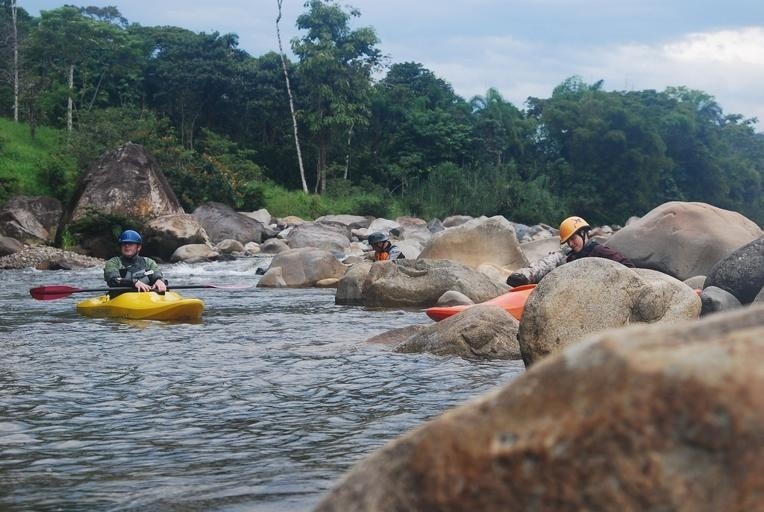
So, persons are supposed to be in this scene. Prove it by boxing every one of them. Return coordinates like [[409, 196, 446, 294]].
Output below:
[[367, 232, 405, 261], [557, 215, 635, 268], [101, 229, 170, 300]]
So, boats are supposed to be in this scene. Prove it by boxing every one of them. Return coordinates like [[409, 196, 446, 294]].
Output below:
[[75, 290, 203, 321], [424, 283, 537, 322]]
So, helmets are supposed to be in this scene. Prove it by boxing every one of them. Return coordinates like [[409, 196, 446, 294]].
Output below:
[[117, 229, 142, 243], [368, 232, 389, 244], [560, 216, 590, 244]]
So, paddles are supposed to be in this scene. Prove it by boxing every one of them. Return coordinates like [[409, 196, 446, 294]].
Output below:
[[30, 284, 255, 300]]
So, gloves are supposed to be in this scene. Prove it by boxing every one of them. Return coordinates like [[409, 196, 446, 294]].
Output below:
[[507, 273, 528, 287]]
